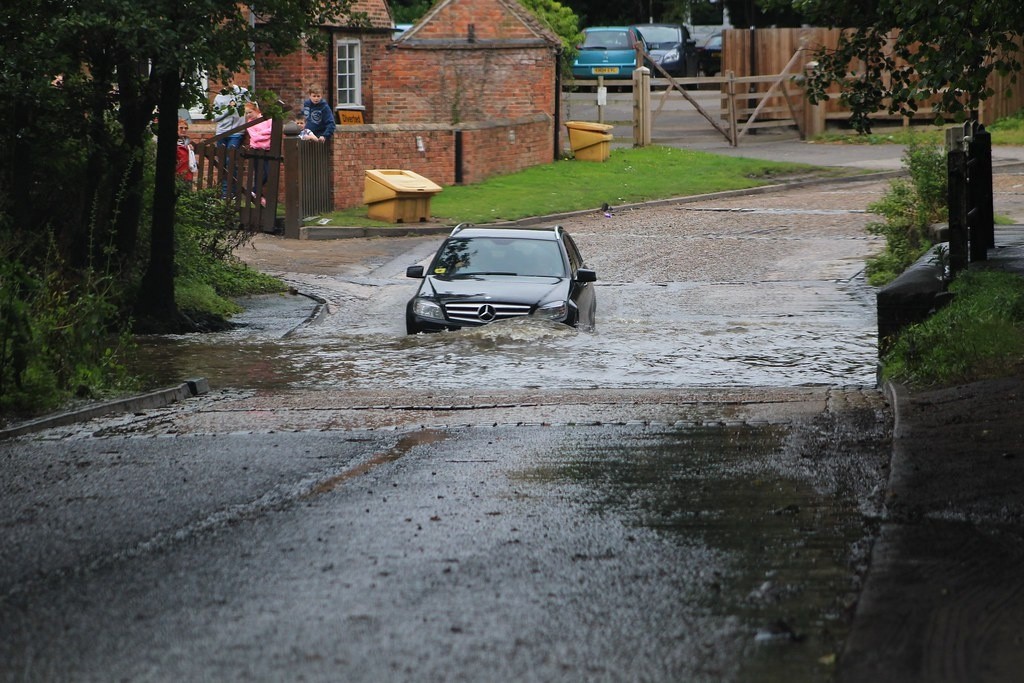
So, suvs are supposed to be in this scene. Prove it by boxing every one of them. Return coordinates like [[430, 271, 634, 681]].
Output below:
[[405, 222, 597, 336]]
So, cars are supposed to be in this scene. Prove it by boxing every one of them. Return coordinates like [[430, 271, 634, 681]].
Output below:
[[634, 24, 699, 92], [569, 26, 654, 94], [690, 31, 723, 77]]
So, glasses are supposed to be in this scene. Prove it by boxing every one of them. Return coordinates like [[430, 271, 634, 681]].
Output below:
[[178, 127, 188, 130]]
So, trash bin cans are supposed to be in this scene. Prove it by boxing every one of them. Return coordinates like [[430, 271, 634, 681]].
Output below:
[[363, 167, 443, 222], [565, 121, 614, 162]]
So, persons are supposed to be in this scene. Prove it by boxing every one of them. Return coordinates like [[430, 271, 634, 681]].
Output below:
[[300, 84, 335, 143], [245, 103, 272, 207], [211, 73, 259, 201], [176, 119, 197, 193], [294, 116, 318, 143]]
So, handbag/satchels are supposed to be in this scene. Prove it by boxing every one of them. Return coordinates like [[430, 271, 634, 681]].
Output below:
[[188, 143, 197, 173]]
